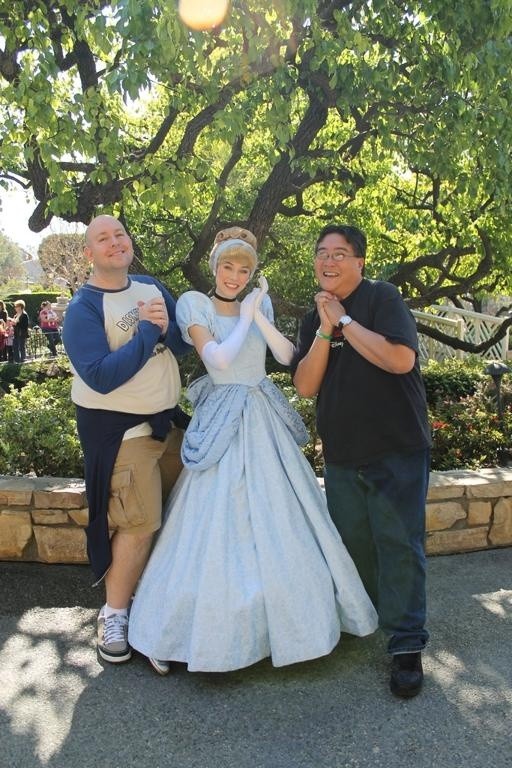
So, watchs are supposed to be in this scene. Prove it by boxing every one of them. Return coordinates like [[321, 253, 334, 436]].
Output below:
[[336, 314, 353, 332]]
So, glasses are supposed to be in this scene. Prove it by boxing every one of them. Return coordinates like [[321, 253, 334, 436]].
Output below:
[[318, 251, 355, 261]]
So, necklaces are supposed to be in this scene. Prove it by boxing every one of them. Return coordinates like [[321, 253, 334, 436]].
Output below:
[[212, 288, 237, 306]]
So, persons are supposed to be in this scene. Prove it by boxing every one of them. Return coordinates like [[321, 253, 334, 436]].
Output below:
[[39, 299, 61, 356], [293, 223, 438, 698], [61, 211, 198, 679], [1, 299, 30, 362], [125, 223, 382, 674]]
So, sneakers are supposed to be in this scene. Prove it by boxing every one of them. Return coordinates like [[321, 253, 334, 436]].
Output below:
[[391, 653, 422, 696], [97, 606, 131, 663]]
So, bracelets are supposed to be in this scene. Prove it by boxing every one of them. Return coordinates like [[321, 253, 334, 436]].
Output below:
[[314, 327, 333, 342]]
